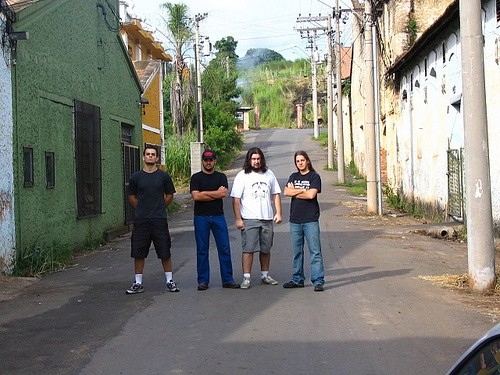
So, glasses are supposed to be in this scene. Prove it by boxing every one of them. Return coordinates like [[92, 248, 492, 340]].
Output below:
[[146, 153, 155, 156], [204, 158, 213, 162]]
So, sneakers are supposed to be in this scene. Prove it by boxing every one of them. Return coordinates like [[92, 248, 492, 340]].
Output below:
[[126, 280, 145, 294], [223, 280, 241, 289], [166, 279, 180, 292], [261, 273, 278, 285], [315, 284, 324, 291], [198, 282, 209, 290], [240, 277, 252, 289], [283, 281, 305, 288]]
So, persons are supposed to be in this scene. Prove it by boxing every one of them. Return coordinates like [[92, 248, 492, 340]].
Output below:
[[126, 146, 179, 293], [190, 150, 240, 291], [283, 150, 326, 291], [230, 146, 284, 289]]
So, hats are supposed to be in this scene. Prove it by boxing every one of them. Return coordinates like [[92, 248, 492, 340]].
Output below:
[[202, 151, 216, 160]]
